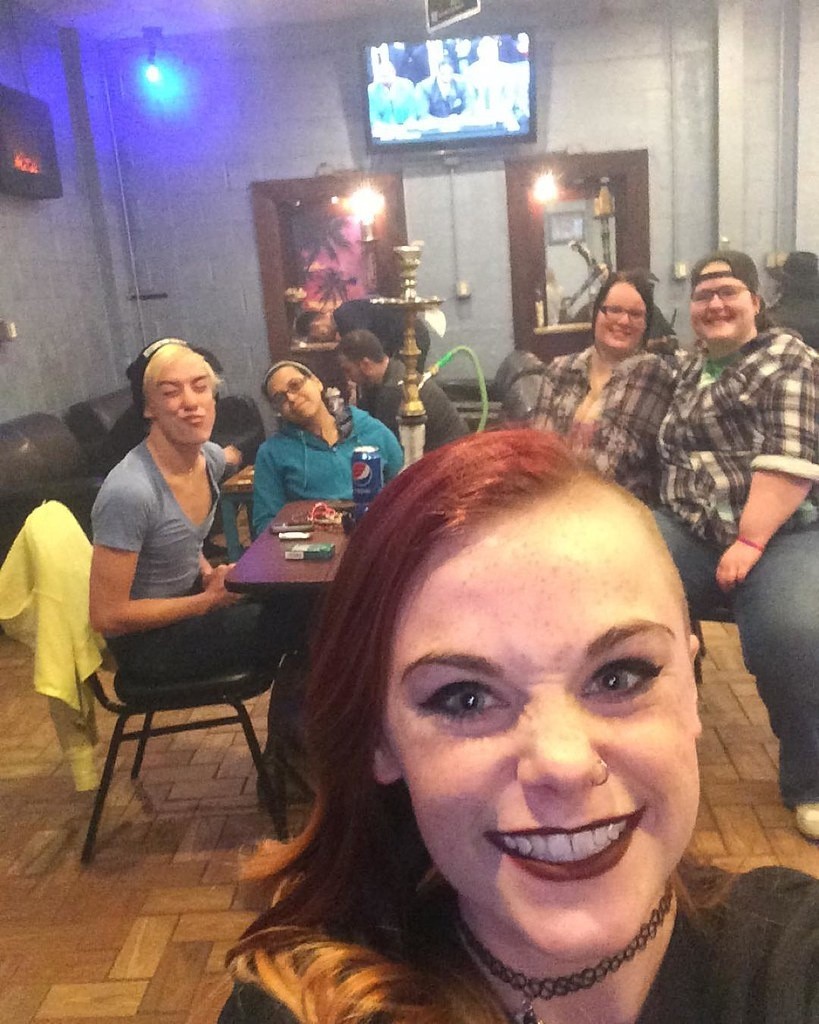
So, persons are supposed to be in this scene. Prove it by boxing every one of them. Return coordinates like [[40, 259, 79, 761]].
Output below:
[[89, 338, 317, 808], [296, 297, 430, 418], [650, 250, 819, 841], [765, 252, 819, 354], [330, 328, 470, 454], [251, 361, 405, 539], [80, 347, 250, 562], [363, 33, 529, 140], [533, 270, 677, 511], [217, 426, 819, 1024]]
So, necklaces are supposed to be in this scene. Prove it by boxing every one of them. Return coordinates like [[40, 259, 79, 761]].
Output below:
[[162, 453, 198, 475], [454, 878, 672, 1024]]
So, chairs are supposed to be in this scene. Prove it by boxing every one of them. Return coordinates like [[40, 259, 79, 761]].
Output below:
[[23, 491, 293, 868]]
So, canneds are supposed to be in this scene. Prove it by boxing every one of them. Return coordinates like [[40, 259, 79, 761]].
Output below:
[[352, 446, 382, 522]]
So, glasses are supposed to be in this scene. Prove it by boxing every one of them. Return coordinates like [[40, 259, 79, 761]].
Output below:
[[273, 374, 310, 411], [598, 304, 646, 322], [690, 283, 749, 305]]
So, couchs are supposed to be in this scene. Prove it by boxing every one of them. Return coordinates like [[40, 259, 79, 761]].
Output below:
[[0, 409, 208, 583], [62, 390, 266, 479]]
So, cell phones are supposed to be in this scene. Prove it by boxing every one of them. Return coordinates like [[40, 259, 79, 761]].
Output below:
[[272, 521, 315, 534]]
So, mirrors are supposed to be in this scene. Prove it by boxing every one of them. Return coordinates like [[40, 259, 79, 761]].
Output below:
[[244, 165, 413, 374], [502, 147, 651, 360]]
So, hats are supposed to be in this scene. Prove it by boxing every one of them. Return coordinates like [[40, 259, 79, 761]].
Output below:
[[766, 252, 819, 291], [691, 250, 758, 293]]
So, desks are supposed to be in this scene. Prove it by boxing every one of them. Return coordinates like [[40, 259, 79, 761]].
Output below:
[[221, 494, 356, 804]]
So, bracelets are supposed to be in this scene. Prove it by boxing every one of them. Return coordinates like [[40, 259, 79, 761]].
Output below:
[[737, 536, 765, 553]]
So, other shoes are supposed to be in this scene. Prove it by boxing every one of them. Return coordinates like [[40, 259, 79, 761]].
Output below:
[[258, 754, 316, 811], [795, 802, 819, 840]]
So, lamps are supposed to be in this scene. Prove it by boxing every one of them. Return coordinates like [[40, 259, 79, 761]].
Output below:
[[139, 26, 163, 85]]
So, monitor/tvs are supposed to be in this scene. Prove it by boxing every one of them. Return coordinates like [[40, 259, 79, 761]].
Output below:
[[360, 30, 537, 154]]
